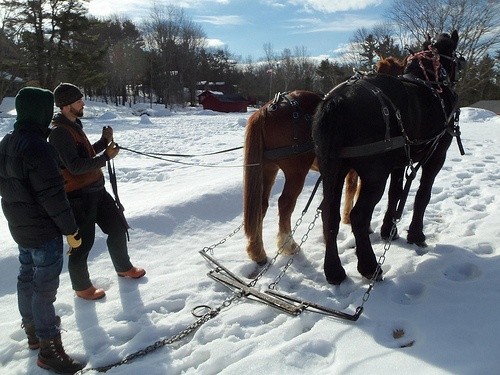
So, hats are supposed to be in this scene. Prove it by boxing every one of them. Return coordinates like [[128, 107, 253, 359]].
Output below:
[[53, 83, 83, 107]]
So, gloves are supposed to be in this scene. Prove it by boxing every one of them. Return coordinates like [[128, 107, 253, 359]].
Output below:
[[66, 228, 82, 256], [102, 126, 113, 143], [104, 142, 119, 160]]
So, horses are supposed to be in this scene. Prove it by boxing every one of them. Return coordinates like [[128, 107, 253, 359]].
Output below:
[[243, 52, 410, 265], [313, 29, 459, 285]]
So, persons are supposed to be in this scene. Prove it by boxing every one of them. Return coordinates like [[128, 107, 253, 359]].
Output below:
[[0, 86, 83, 374], [50, 82, 145, 299]]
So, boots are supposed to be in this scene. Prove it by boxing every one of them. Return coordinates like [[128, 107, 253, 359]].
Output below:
[[21, 315, 61, 350], [37, 334, 82, 375]]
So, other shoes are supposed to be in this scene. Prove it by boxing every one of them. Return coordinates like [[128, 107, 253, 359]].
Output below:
[[117, 266, 146, 278], [75, 285, 105, 300]]
